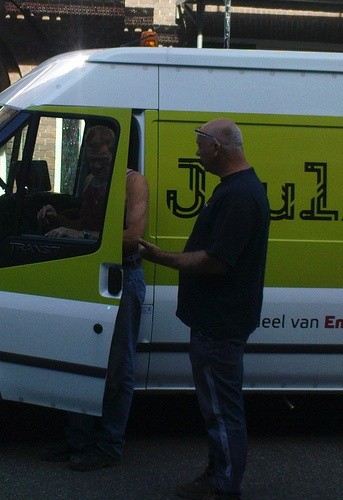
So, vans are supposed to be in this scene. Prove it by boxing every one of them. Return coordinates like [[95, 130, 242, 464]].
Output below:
[[0, 46, 343, 417]]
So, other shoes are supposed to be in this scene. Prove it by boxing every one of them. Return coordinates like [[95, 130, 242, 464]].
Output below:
[[69, 454, 123, 469], [40, 442, 82, 461], [177, 480, 243, 500]]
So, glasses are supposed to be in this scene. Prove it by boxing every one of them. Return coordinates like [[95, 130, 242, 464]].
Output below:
[[195, 127, 213, 138]]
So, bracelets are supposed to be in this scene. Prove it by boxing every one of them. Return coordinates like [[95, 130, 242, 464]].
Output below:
[[82, 228, 91, 239]]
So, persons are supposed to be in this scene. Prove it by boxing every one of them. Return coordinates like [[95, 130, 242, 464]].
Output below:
[[138, 118, 272, 500], [36, 125, 150, 472]]
